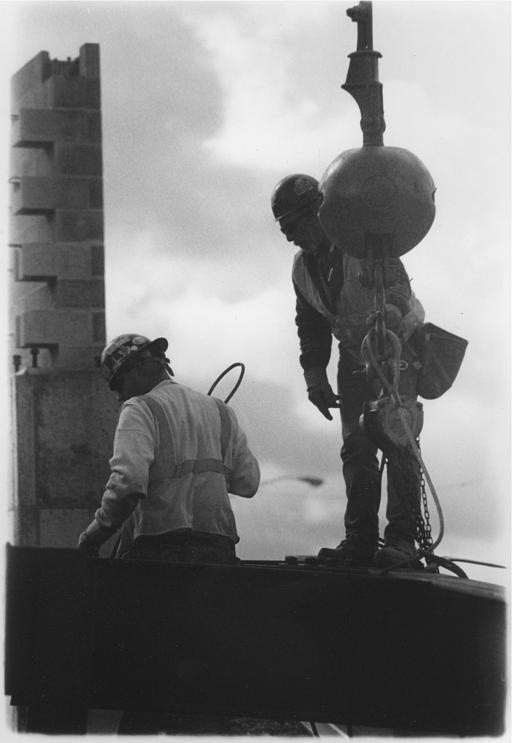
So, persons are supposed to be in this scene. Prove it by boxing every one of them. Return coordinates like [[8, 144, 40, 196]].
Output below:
[[73, 328, 263, 562], [271, 173, 432, 569]]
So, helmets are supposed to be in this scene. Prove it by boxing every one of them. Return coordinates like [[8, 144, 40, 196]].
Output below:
[[100, 333, 168, 391], [271, 174, 321, 223]]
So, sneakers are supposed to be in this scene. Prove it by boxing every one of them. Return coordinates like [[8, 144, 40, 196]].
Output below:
[[318, 541, 370, 566], [374, 538, 419, 569]]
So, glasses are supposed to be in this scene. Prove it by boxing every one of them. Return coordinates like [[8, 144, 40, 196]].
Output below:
[[280, 213, 309, 234]]
